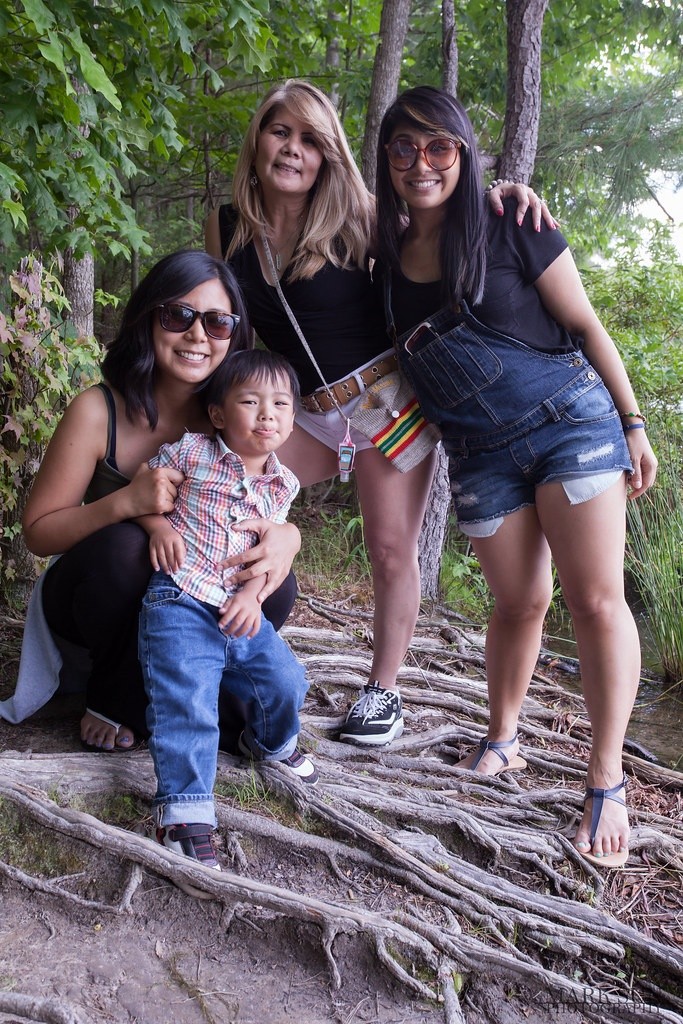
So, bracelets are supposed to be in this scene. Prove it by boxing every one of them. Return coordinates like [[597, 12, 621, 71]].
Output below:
[[483, 179, 514, 196], [620, 412, 646, 422], [623, 423, 644, 431]]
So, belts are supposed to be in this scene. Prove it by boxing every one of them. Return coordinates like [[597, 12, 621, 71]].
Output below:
[[301, 352, 400, 413]]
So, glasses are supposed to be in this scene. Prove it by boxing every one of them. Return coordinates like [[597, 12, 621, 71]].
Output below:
[[155, 302, 240, 340], [384, 138, 461, 171]]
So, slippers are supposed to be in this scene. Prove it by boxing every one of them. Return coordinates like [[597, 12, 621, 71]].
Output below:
[[82, 707, 136, 751]]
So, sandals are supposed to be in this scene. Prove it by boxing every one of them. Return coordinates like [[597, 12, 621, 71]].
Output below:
[[573, 771, 628, 868], [452, 733, 527, 777]]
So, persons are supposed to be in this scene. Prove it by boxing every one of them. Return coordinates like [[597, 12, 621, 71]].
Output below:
[[0, 248, 320, 874], [203, 77, 660, 868]]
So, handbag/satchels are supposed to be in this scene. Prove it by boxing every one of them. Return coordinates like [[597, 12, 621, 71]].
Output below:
[[348, 369, 441, 474]]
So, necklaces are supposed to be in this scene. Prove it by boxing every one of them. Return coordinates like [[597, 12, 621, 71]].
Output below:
[[270, 211, 304, 272]]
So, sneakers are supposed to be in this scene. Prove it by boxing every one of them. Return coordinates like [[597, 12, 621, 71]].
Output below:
[[152, 821, 223, 899], [340, 680, 404, 747], [238, 729, 319, 787]]
